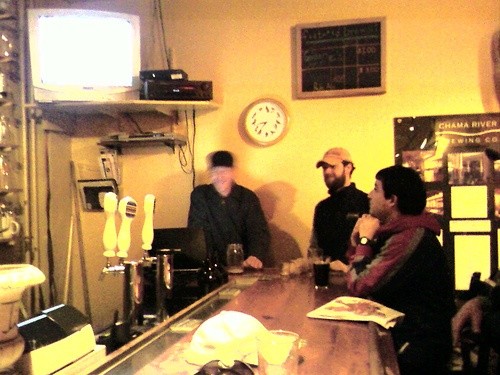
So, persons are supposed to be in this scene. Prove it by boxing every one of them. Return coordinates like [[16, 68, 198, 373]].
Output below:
[[187, 150, 272, 269], [346, 166, 455, 375], [452, 268, 500, 375], [306, 147, 370, 268]]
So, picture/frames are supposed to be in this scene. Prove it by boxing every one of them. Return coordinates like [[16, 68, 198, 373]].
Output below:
[[295, 14, 386, 99]]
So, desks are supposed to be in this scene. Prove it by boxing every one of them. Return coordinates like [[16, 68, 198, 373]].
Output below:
[[79, 267, 399, 375]]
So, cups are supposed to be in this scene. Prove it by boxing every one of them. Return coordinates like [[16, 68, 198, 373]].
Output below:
[[307, 248, 323, 266], [256, 330, 300, 375], [313, 263, 330, 291]]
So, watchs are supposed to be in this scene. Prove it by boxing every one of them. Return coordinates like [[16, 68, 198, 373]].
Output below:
[[359, 236, 376, 249]]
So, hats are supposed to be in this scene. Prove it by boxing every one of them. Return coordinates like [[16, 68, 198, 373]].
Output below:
[[186, 309, 293, 366], [316, 147, 353, 169]]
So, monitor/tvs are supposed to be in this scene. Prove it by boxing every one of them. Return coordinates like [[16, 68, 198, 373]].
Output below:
[[25, 8, 141, 103]]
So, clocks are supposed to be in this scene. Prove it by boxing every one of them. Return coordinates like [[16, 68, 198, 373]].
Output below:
[[244, 98, 291, 148]]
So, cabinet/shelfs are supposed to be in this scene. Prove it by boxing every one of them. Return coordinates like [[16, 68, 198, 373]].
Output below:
[[24, 99, 220, 154]]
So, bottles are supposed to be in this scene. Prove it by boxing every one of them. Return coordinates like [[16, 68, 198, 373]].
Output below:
[[226, 243, 244, 273]]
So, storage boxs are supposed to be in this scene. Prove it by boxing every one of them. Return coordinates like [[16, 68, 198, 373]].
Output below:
[[19, 323, 105, 375]]
